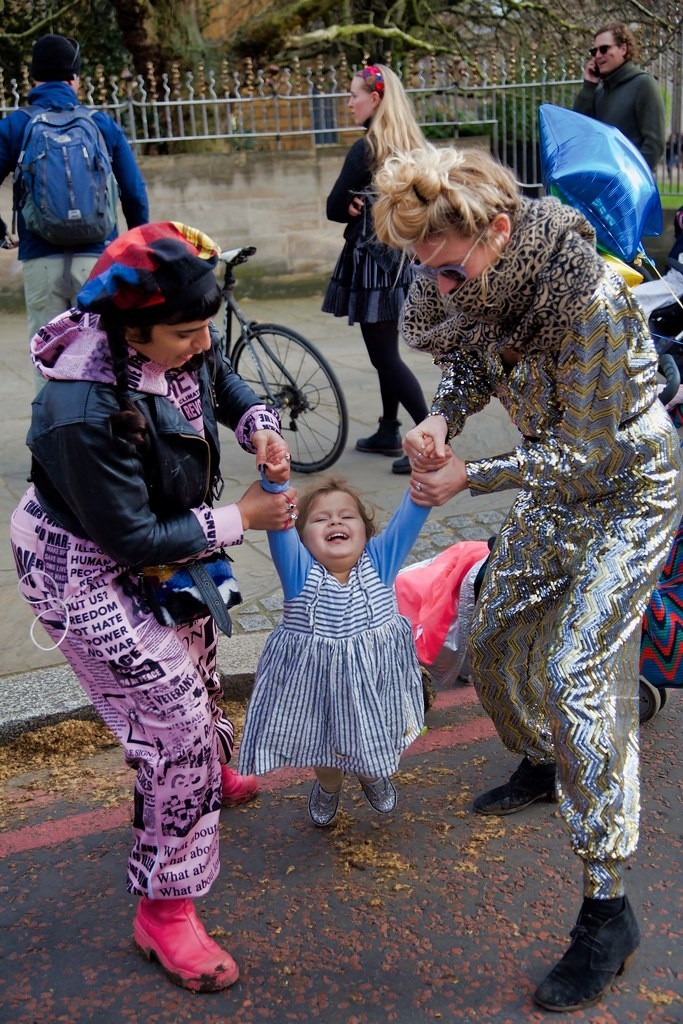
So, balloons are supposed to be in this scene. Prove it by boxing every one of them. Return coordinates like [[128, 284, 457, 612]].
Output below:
[[599, 252, 644, 289], [538, 103, 664, 261]]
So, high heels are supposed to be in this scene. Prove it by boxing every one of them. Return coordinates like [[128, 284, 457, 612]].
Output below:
[[534, 896, 640, 1011], [473, 757, 557, 815]]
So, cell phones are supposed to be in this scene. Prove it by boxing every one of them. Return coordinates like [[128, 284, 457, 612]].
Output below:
[[353, 191, 367, 212], [590, 64, 601, 76]]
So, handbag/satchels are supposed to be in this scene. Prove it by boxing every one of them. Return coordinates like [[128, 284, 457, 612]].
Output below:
[[138, 554, 243, 638]]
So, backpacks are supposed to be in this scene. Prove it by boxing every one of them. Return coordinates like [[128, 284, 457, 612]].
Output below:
[[12, 105, 121, 298]]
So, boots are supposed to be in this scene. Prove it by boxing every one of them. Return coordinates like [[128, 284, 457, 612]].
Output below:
[[392, 455, 412, 473], [356, 417, 404, 457]]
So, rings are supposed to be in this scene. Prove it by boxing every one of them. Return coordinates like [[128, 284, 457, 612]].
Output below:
[[290, 513, 299, 521], [416, 453, 422, 460], [413, 457, 417, 465], [415, 483, 421, 492], [288, 503, 297, 511], [285, 452, 292, 462]]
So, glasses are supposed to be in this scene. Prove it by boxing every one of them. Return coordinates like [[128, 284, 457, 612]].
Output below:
[[409, 226, 489, 283], [66, 37, 79, 67], [589, 44, 624, 57]]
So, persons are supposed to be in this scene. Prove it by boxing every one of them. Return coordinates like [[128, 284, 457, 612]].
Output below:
[[243, 432, 454, 825], [0, 35, 154, 402], [366, 147, 683, 1011], [321, 62, 452, 475], [6, 222, 300, 992], [569, 23, 664, 174]]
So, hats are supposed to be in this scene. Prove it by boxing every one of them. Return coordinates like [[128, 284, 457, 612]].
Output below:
[[77, 221, 222, 313], [31, 34, 84, 82]]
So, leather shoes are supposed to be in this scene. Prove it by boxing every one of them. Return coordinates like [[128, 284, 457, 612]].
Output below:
[[221, 765, 260, 807], [132, 896, 239, 991]]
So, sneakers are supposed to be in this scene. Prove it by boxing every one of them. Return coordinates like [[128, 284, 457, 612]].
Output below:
[[354, 769, 397, 814], [308, 771, 346, 826]]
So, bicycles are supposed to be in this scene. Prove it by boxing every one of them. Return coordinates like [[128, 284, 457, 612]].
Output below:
[[204, 245, 349, 475]]
